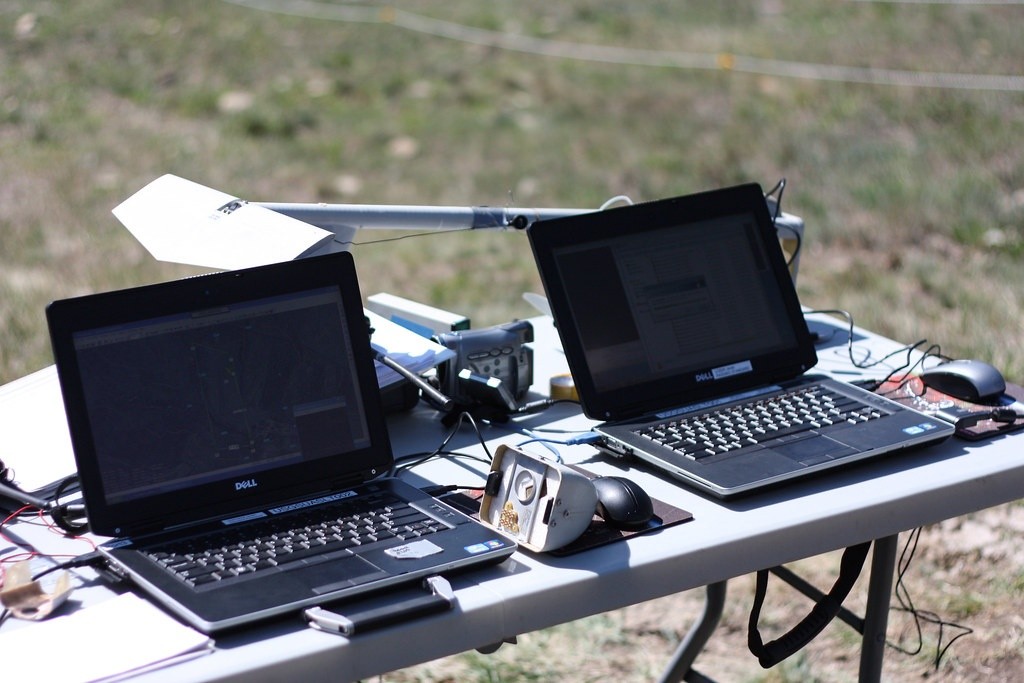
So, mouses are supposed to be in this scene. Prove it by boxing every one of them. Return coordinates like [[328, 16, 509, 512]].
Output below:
[[920, 359, 1008, 403], [587, 475, 653, 529]]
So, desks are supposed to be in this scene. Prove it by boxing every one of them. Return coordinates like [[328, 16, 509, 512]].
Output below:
[[1, 300, 1024, 683]]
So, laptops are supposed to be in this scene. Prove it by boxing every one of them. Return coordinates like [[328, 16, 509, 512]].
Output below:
[[526, 181, 956, 502], [44, 250, 519, 641]]
